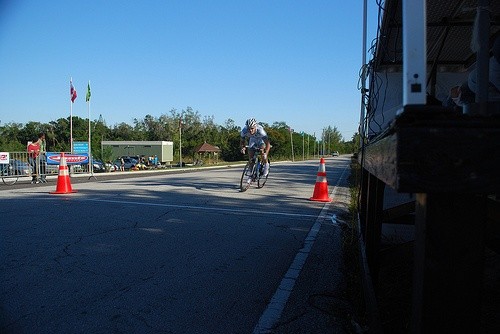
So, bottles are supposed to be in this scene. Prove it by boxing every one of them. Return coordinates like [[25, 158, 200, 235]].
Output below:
[[258, 158, 260, 169]]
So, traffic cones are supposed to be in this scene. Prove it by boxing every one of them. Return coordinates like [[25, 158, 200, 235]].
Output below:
[[50, 152, 78, 194], [309, 157, 331, 202]]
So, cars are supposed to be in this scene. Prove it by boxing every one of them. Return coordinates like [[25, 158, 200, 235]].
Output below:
[[0, 159, 33, 175], [43, 158, 137, 172], [332, 151, 339, 157]]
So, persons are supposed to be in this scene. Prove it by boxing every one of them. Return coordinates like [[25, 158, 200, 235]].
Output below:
[[29, 133, 47, 182], [120, 158, 125, 171], [241, 118, 271, 183]]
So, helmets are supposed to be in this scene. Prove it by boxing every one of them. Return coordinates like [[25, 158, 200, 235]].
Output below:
[[245, 118, 257, 127]]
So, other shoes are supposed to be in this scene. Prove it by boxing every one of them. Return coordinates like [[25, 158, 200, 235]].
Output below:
[[42, 180, 48, 183], [247, 178, 252, 184], [263, 168, 268, 177]]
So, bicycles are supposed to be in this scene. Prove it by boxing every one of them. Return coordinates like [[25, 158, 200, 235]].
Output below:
[[0, 158, 19, 185], [240, 145, 271, 191]]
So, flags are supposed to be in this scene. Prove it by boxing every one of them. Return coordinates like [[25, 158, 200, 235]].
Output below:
[[70, 80, 76, 102], [86, 80, 91, 103]]
